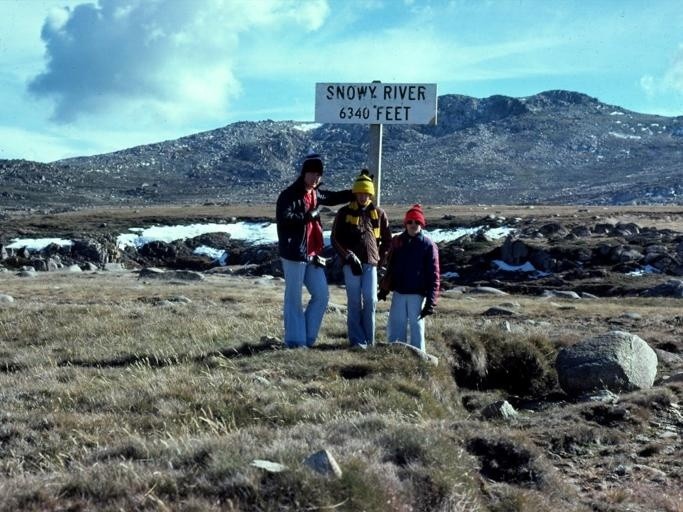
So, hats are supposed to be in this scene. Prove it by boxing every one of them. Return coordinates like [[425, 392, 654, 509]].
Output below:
[[301, 154, 323, 176], [403, 204, 426, 228], [352, 174, 374, 197], [407, 219, 420, 225]]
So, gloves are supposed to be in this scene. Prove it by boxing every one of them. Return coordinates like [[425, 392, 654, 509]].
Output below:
[[419, 303, 434, 318], [309, 205, 323, 220], [377, 288, 389, 302], [347, 252, 363, 276]]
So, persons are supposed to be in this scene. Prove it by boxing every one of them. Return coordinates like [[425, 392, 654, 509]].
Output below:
[[377, 204, 441, 351], [330, 169, 392, 350], [276, 159, 374, 349]]
